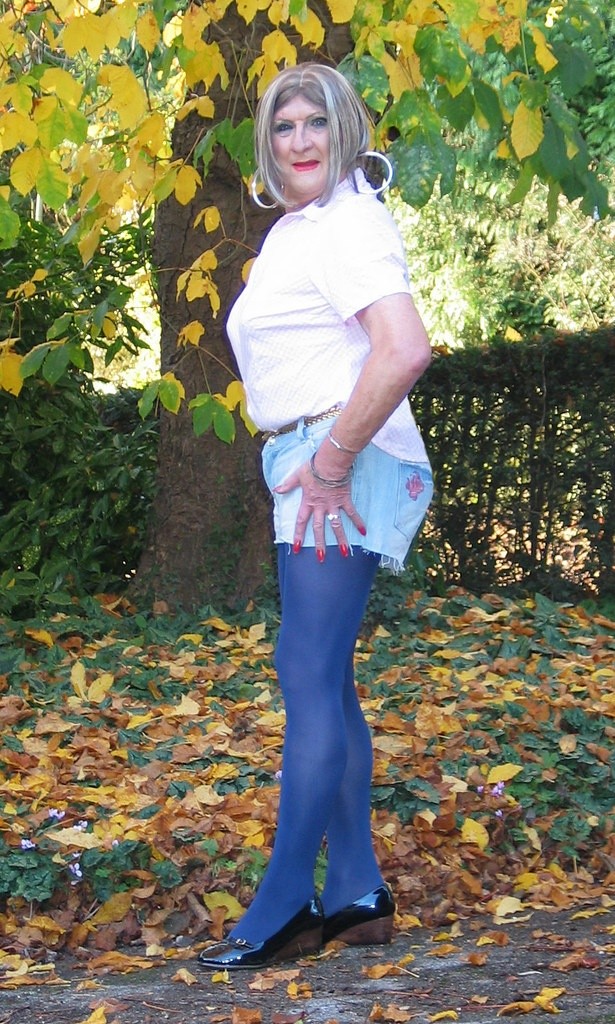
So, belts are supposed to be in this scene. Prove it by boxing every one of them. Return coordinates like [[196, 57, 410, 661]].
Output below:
[[261, 406, 343, 439]]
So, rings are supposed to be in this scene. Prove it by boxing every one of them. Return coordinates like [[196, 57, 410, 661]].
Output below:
[[327, 513, 341, 520]]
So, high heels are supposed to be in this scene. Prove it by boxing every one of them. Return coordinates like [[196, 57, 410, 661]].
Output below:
[[197, 893, 325, 967], [325, 884, 395, 943]]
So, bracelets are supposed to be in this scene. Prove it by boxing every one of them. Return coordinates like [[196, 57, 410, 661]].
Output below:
[[328, 430, 361, 457], [309, 452, 354, 487]]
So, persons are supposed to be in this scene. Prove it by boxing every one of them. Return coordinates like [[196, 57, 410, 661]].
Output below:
[[194, 59, 437, 970]]
